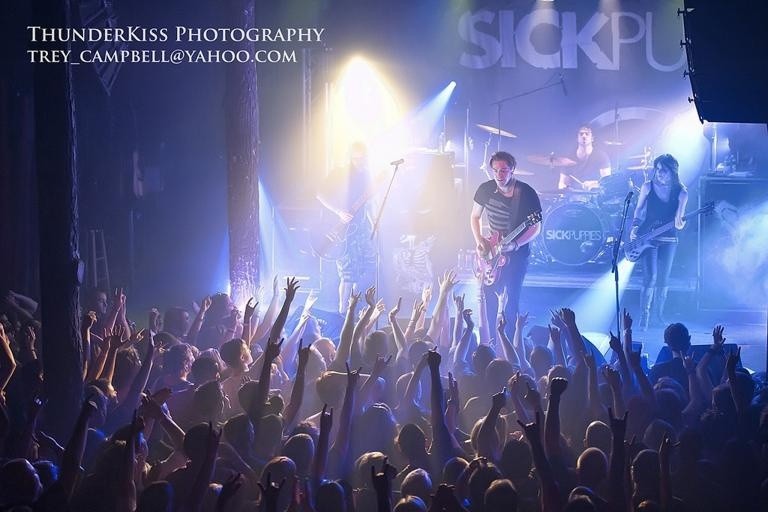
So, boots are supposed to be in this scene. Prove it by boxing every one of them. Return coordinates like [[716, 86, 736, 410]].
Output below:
[[639, 287, 653, 332], [651, 286, 670, 329]]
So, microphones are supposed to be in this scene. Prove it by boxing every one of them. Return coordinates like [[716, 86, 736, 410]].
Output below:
[[559, 74, 567, 96], [625, 191, 634, 204], [390, 158, 404, 166]]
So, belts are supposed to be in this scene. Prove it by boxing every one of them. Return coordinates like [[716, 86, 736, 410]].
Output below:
[[649, 236, 679, 243]]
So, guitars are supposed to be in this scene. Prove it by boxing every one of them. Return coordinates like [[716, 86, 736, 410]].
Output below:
[[473, 209, 541, 286], [624, 201, 716, 262], [309, 186, 374, 258]]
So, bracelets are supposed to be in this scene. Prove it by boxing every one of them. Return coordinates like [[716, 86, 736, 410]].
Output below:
[[631, 218, 641, 227], [513, 239, 519, 252], [337, 208, 341, 214]]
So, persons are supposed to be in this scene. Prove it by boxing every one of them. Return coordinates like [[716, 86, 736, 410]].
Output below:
[[0, 268, 768, 512], [470, 151, 542, 347], [629, 154, 689, 332], [316, 139, 377, 320], [559, 123, 610, 197]]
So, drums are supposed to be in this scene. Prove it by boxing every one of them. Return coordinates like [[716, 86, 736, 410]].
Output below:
[[598, 171, 638, 204], [542, 201, 608, 264]]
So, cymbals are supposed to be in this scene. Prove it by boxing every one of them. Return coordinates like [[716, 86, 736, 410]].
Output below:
[[628, 166, 654, 169], [602, 142, 628, 145], [526, 154, 577, 165], [514, 170, 534, 175], [538, 187, 598, 195], [477, 124, 516, 138], [632, 155, 653, 158]]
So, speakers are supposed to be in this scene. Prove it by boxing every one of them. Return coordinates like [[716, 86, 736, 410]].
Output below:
[[392, 316, 455, 338], [676, 0, 768, 125], [654, 343, 743, 386], [285, 305, 346, 348], [271, 215, 323, 294], [526, 324, 607, 368]]
